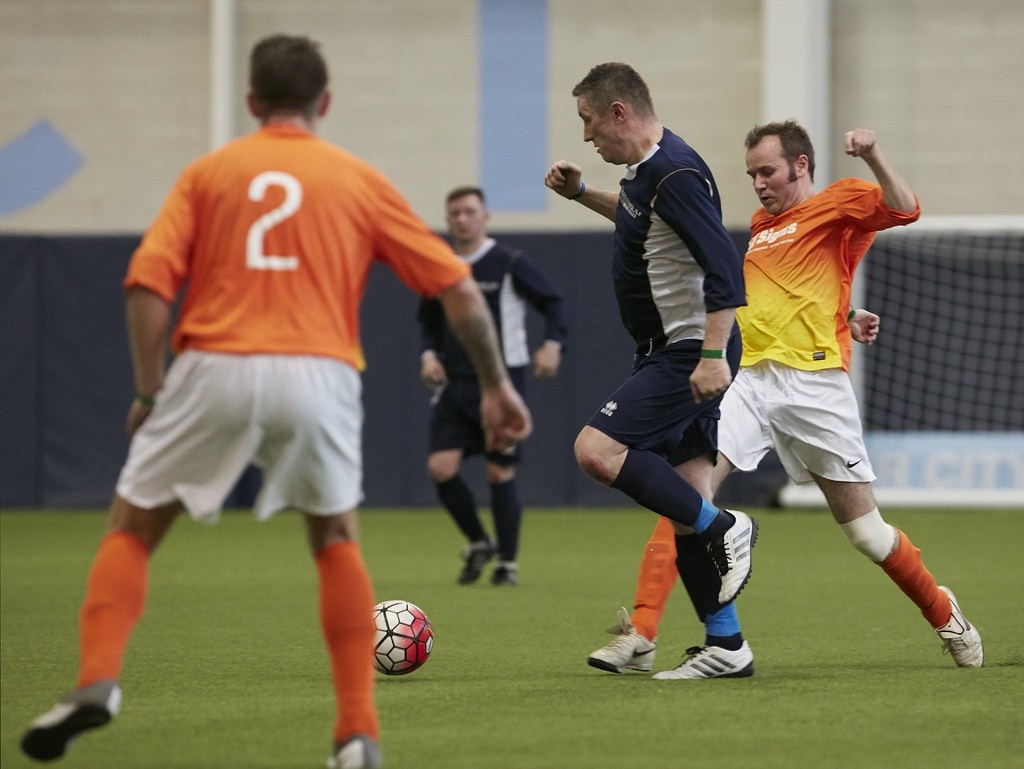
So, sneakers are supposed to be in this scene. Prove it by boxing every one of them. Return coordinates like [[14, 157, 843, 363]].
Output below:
[[494, 565, 520, 587], [705, 507, 759, 605], [935, 584, 984, 668], [327, 736, 380, 768], [19, 684, 122, 762], [460, 544, 495, 584], [587, 604, 655, 671], [653, 639, 754, 680]]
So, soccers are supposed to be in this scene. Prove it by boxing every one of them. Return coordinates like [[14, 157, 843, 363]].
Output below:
[[372, 600, 435, 675]]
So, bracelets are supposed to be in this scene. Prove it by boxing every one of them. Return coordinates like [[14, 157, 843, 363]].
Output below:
[[847, 305, 856, 319], [700, 348, 726, 359], [138, 391, 154, 405]]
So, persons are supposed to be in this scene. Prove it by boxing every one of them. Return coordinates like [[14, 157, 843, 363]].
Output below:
[[18, 37, 530, 769], [545, 62, 756, 680], [586, 117, 986, 674], [419, 186, 564, 591]]
